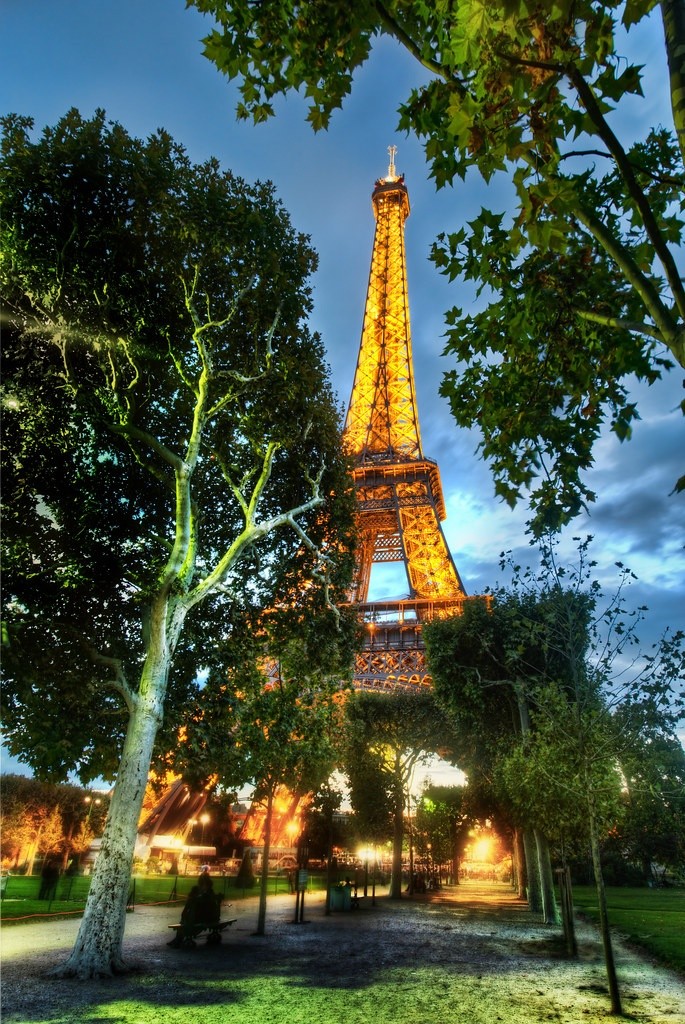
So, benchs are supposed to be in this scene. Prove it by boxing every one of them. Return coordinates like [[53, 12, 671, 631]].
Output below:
[[351, 897, 365, 910], [166, 919, 237, 946]]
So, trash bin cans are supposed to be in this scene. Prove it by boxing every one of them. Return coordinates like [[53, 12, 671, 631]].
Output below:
[[329, 885, 352, 913]]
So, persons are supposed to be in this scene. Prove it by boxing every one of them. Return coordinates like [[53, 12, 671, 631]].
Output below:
[[198, 866, 213, 889], [164, 886, 224, 948]]
